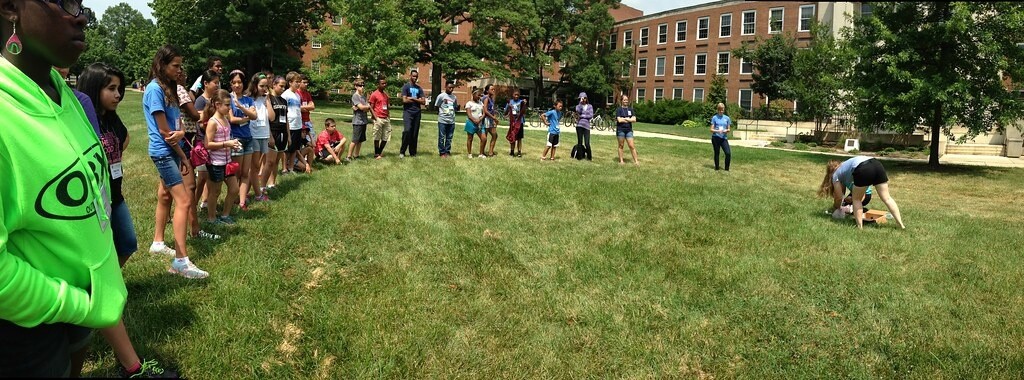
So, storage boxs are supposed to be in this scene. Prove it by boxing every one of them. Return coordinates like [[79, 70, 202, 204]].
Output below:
[[851, 210, 888, 224]]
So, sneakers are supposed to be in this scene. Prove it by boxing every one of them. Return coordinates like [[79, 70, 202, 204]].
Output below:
[[147, 243, 176, 258], [167, 261, 209, 280], [126, 360, 180, 380]]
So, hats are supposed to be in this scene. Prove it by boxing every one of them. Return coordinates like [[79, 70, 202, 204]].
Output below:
[[578, 92, 587, 98]]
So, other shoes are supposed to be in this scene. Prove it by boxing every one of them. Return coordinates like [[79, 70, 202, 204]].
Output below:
[[207, 218, 229, 228], [398, 153, 556, 162], [165, 218, 174, 223], [244, 186, 279, 206], [374, 154, 388, 161], [345, 155, 364, 163], [219, 217, 236, 225], [281, 169, 296, 177], [238, 204, 249, 213], [190, 230, 221, 240], [198, 205, 219, 214]]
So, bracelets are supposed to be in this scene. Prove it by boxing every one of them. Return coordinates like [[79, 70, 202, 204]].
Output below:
[[223, 142, 224, 148]]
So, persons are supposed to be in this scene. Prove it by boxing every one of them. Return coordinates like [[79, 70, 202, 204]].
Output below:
[[400, 70, 425, 158], [53, 67, 178, 380], [166, 57, 315, 238], [142, 45, 210, 279], [77, 63, 137, 269], [464, 86, 487, 158], [541, 100, 563, 161], [368, 79, 391, 159], [504, 90, 525, 156], [0, 0, 127, 379], [347, 79, 371, 160], [576, 92, 593, 161], [616, 95, 639, 166], [710, 103, 731, 171], [817, 156, 905, 229], [481, 84, 499, 156], [315, 117, 346, 164], [140, 77, 144, 91], [435, 82, 457, 157]]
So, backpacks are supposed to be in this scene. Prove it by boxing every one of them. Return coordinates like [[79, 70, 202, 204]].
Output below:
[[570, 144, 585, 160]]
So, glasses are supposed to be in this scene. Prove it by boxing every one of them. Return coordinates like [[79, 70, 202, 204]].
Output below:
[[48, 0, 92, 29], [357, 84, 365, 88]]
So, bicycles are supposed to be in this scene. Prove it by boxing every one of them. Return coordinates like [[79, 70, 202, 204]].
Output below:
[[526, 106, 617, 132], [493, 108, 510, 122]]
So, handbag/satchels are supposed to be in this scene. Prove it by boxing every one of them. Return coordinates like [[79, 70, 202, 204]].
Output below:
[[189, 140, 212, 168], [225, 162, 242, 176]]
